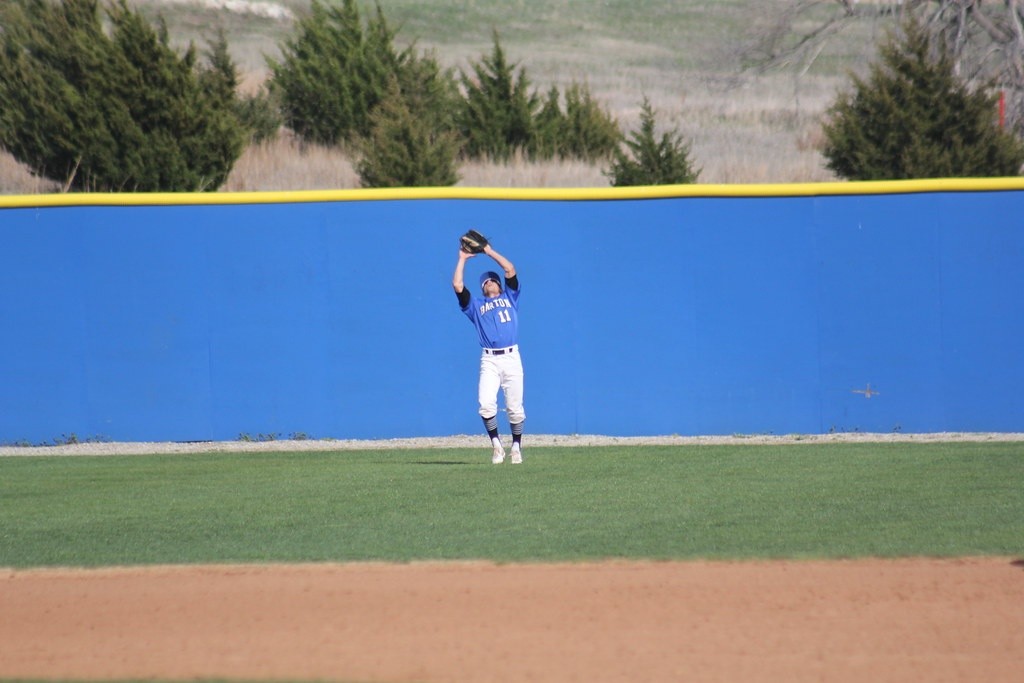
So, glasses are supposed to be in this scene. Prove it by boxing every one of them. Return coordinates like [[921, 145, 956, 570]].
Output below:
[[484, 280, 500, 287]]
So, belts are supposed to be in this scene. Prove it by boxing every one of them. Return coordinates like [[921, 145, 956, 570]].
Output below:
[[486, 348, 512, 355]]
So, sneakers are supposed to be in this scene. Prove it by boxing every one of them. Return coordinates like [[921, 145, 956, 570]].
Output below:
[[491, 438, 505, 463], [510, 442, 522, 464]]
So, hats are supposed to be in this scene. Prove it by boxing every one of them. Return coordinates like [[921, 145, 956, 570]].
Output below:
[[480, 271, 502, 291]]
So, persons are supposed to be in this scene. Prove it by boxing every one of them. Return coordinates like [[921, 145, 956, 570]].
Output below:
[[452, 245, 526, 465]]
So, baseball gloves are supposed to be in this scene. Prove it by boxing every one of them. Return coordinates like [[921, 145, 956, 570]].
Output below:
[[459, 229, 487, 254]]
